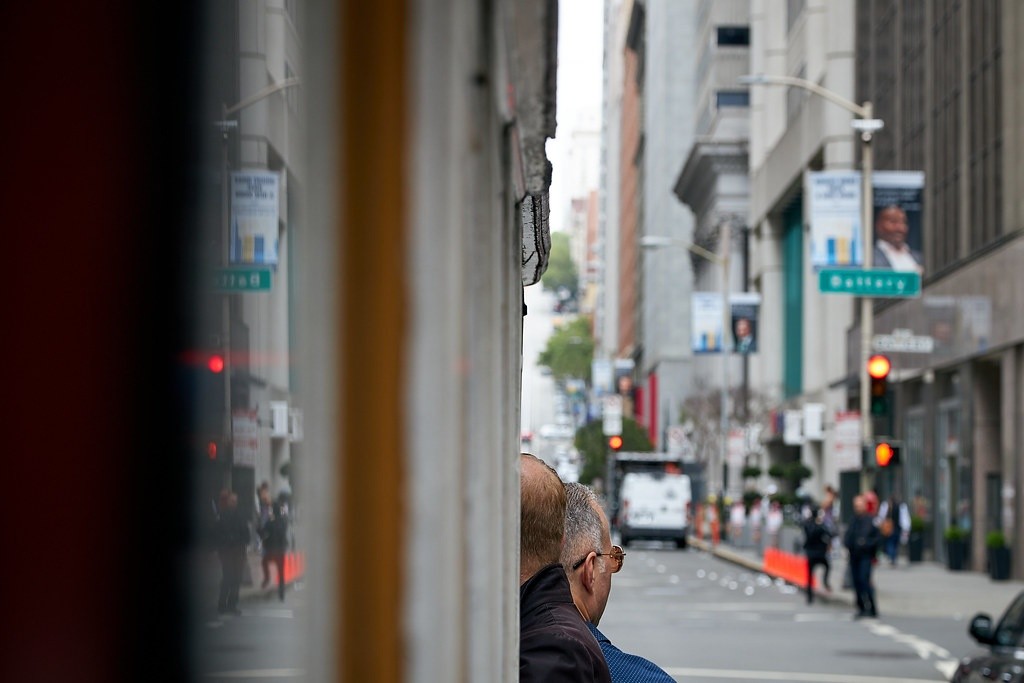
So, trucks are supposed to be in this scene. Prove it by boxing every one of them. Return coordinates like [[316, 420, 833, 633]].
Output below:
[[609, 451, 690, 550]]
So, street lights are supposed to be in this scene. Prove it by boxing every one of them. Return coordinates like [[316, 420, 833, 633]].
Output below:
[[741, 74, 877, 498], [638, 237, 730, 498], [214, 73, 305, 471]]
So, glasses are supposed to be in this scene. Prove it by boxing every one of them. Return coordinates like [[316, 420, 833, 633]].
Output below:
[[573, 543, 626, 575]]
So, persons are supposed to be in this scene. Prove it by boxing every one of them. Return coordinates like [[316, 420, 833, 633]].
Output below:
[[218, 482, 289, 615], [616, 373, 634, 400], [734, 318, 756, 353], [872, 204, 926, 273], [800, 484, 839, 603], [517, 452, 612, 683], [844, 489, 912, 619], [728, 491, 785, 558], [562, 481, 678, 683]]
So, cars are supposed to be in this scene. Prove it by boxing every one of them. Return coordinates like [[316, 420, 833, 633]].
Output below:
[[950, 590, 1024, 683]]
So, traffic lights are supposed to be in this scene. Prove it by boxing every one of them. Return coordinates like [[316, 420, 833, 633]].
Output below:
[[203, 443, 220, 465], [867, 353, 892, 417], [609, 436, 624, 451], [205, 358, 227, 384], [873, 441, 902, 470]]
